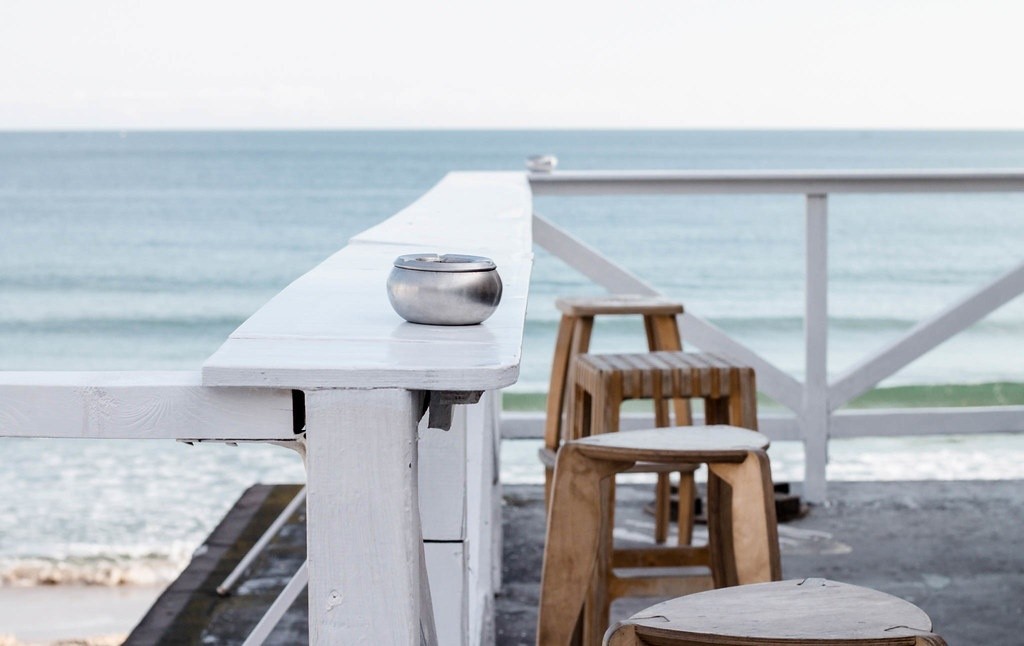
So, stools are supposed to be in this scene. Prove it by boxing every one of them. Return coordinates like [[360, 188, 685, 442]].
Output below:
[[536, 295, 697, 548], [561, 352, 757, 438], [540, 420, 786, 645], [603, 575, 951, 646]]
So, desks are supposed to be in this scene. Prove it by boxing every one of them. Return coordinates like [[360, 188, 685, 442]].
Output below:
[[205, 166, 1022, 645]]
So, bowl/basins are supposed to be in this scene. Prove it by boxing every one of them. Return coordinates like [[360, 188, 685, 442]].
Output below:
[[526, 155, 556, 171], [386, 254, 503, 325]]
